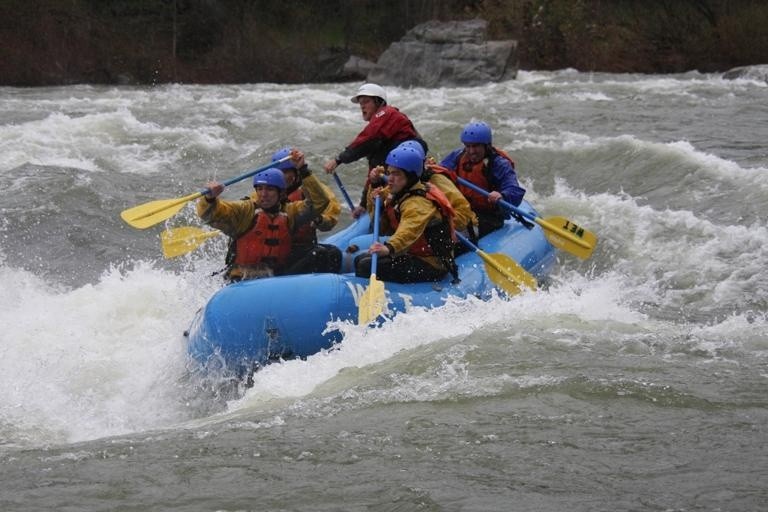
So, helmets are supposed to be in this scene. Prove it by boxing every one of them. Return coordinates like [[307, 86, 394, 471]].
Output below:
[[384, 147, 424, 179], [398, 140, 428, 161], [269, 146, 303, 170], [460, 118, 493, 145], [252, 164, 288, 190], [350, 83, 388, 104]]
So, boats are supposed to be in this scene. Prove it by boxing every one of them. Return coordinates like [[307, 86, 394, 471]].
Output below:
[[181, 198, 558, 387]]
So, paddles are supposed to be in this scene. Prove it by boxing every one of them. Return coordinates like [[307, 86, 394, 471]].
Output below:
[[160, 227, 222, 257], [120, 155, 293, 229], [428, 158, 596, 259], [380, 174, 538, 298], [359, 195, 384, 327]]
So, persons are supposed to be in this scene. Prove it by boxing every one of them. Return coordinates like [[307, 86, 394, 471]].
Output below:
[[355, 147, 458, 283], [366, 139, 479, 255], [324, 82, 428, 218], [197, 148, 330, 283], [440, 121, 525, 237], [240, 146, 342, 274]]
